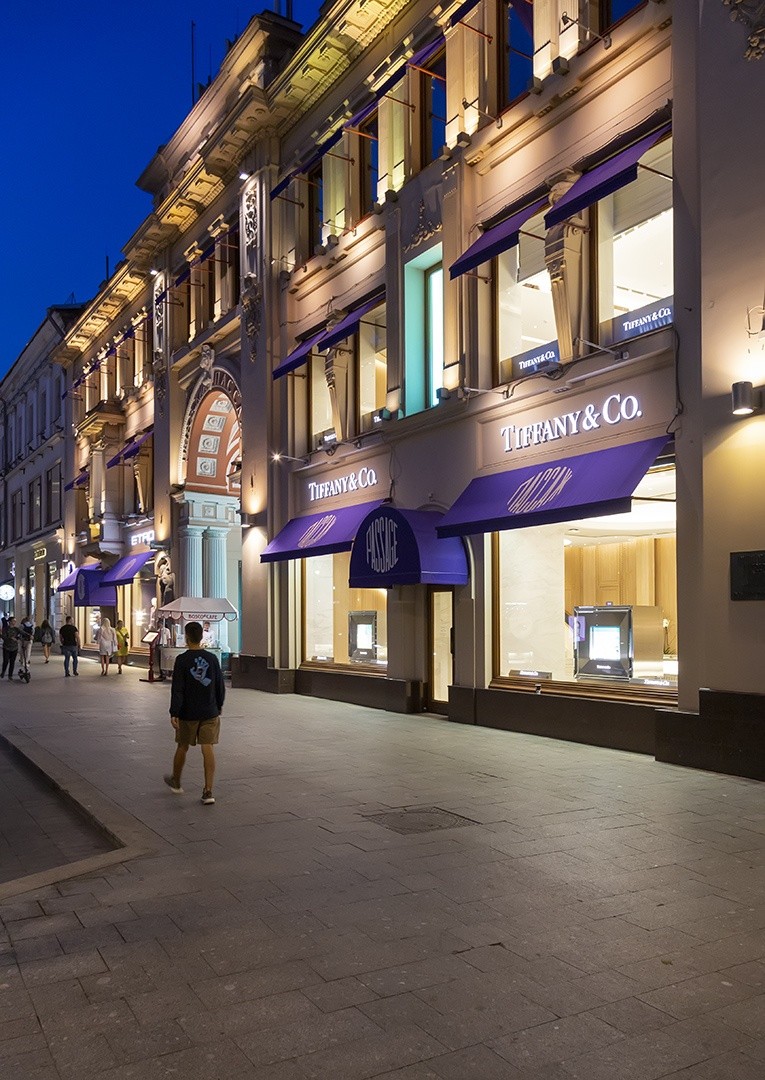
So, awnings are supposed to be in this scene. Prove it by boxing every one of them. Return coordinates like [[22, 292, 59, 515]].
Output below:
[[432, 434, 670, 540], [448, 191, 551, 283], [259, 498, 392, 563], [106, 430, 153, 468], [61, 218, 239, 400], [349, 507, 468, 586], [99, 552, 154, 588], [272, 293, 385, 381], [74, 569, 118, 607], [57, 562, 102, 592], [64, 469, 89, 492], [269, 0, 483, 203], [544, 118, 672, 227]]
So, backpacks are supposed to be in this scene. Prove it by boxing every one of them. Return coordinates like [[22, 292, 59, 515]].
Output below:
[[21, 623, 33, 641], [42, 631, 52, 643]]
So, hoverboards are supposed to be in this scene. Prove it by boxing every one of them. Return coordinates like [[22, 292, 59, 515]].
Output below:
[[14, 635, 32, 684]]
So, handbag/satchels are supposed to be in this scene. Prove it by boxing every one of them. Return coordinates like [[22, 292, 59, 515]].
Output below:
[[125, 642, 128, 647]]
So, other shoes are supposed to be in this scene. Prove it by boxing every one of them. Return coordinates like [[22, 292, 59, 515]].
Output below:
[[73, 672, 79, 675], [65, 674, 70, 677]]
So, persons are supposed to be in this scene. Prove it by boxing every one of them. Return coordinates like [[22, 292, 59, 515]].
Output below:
[[96, 617, 129, 676], [163, 622, 225, 806], [59, 616, 79, 677], [202, 621, 214, 648], [1, 613, 34, 680], [155, 620, 171, 682], [39, 620, 55, 663]]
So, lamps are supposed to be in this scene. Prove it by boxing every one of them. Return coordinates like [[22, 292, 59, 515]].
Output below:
[[318, 222, 357, 236], [458, 386, 508, 401], [572, 335, 624, 362], [561, 11, 612, 49], [462, 98, 503, 128], [269, 258, 307, 272], [732, 381, 762, 416]]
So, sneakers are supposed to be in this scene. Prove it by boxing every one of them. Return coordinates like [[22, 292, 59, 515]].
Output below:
[[163, 774, 184, 794], [200, 788, 215, 805]]
